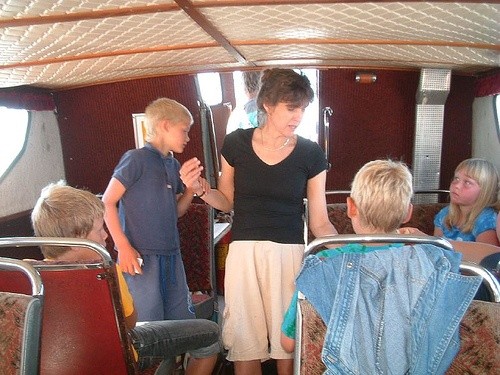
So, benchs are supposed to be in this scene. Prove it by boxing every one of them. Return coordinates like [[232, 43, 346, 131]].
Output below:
[[293, 189, 500, 375], [101, 199, 215, 324]]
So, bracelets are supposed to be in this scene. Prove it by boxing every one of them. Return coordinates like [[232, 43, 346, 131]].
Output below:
[[193, 191, 205, 198]]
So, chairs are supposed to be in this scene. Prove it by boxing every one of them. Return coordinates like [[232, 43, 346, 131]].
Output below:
[[0, 237, 137, 375]]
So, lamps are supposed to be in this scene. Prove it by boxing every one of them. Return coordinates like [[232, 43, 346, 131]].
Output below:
[[354, 72, 377, 84]]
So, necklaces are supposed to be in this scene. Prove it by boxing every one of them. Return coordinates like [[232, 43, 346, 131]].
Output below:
[[260, 128, 290, 151]]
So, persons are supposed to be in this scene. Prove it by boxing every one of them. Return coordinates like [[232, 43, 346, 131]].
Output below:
[[397, 213, 500, 302], [178, 68, 346, 375], [102, 97, 211, 321], [280, 159, 413, 353], [31, 179, 222, 375], [433, 158, 499, 246]]
